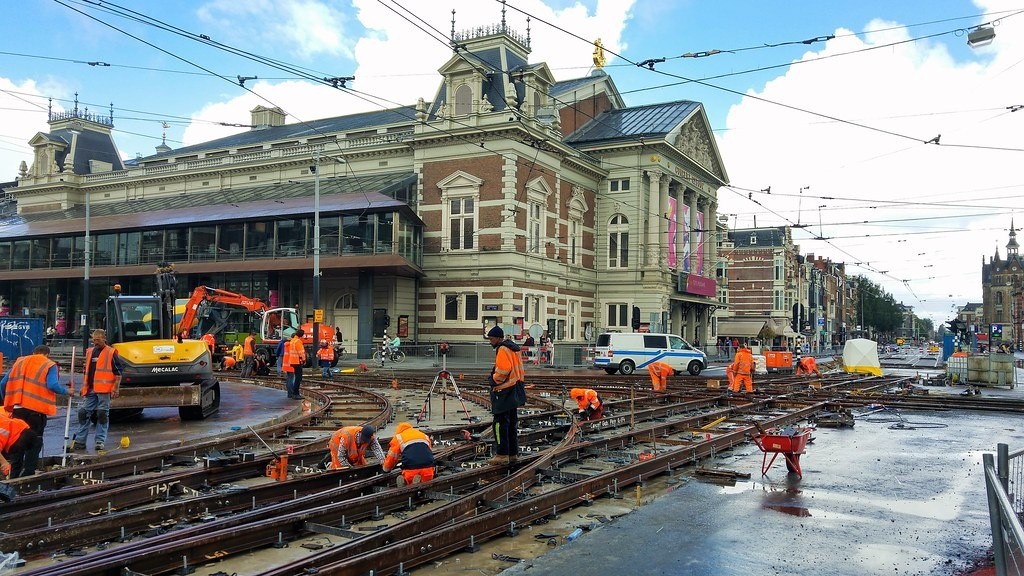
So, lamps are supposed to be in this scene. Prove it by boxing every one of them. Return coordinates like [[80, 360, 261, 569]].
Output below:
[[720, 215, 728, 222], [967, 26, 996, 46], [728, 259, 734, 265], [537, 106, 557, 122], [65, 121, 83, 134]]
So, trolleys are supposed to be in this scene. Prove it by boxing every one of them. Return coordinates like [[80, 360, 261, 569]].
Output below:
[[744, 427, 811, 479]]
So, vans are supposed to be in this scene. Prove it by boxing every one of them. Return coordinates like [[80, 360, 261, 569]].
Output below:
[[592, 332, 708, 376]]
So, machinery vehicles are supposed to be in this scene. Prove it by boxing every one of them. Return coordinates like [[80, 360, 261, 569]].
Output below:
[[90, 260, 221, 425], [177, 285, 340, 368]]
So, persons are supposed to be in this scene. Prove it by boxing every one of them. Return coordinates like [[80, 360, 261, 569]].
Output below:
[[570, 388, 604, 420], [46, 317, 66, 339], [691, 337, 839, 393], [326, 425, 386, 470], [382, 422, 434, 486], [385, 333, 400, 355], [484, 326, 527, 464], [648, 363, 681, 392], [275, 327, 343, 400], [63, 329, 123, 449], [199, 332, 271, 379], [0, 345, 74, 480], [506, 332, 555, 366], [439, 342, 450, 355]]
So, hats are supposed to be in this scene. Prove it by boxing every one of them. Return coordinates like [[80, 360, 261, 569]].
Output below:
[[487, 326, 504, 338], [361, 425, 374, 442]]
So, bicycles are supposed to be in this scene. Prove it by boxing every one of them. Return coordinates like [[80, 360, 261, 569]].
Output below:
[[338, 344, 348, 361], [423, 337, 449, 357], [373, 342, 405, 364]]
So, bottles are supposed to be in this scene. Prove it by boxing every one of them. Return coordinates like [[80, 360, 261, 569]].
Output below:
[[567, 528, 583, 541]]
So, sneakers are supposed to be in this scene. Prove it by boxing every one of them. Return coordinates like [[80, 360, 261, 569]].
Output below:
[[486, 454, 518, 465], [63, 440, 86, 450], [95, 441, 104, 450]]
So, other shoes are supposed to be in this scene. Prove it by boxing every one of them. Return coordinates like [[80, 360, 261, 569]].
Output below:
[[727, 390, 732, 397], [412, 475, 421, 485], [317, 452, 331, 469], [396, 475, 405, 487], [292, 394, 305, 399], [19, 481, 38, 495]]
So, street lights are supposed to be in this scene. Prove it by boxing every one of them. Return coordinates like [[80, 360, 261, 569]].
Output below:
[[310, 148, 346, 370]]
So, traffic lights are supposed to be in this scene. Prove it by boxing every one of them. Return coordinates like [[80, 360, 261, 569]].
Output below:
[[945, 320, 958, 336], [958, 320, 967, 333]]
